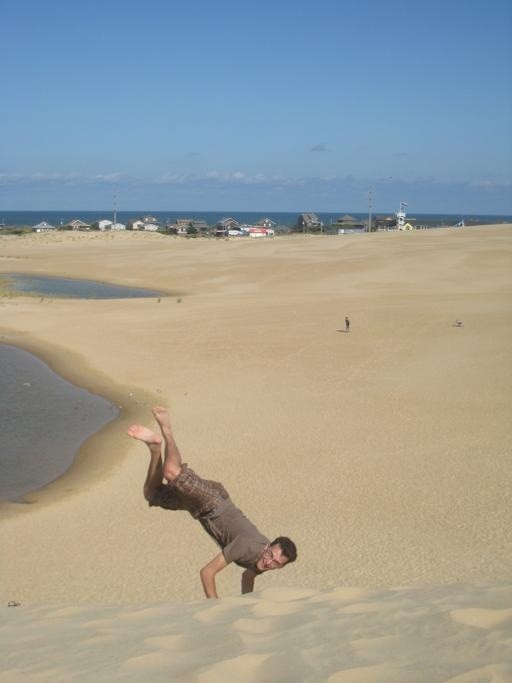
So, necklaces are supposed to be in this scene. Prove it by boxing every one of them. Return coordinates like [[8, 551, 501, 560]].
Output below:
[[127, 407, 297, 599]]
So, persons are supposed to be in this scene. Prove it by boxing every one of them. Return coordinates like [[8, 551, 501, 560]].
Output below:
[[344, 315, 350, 333]]
